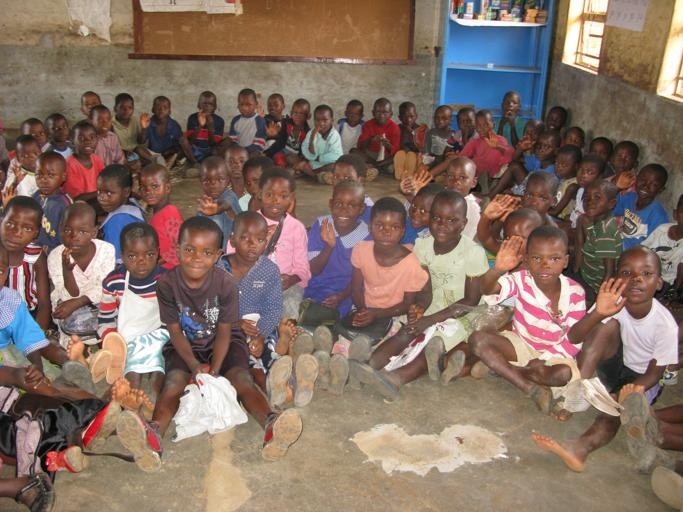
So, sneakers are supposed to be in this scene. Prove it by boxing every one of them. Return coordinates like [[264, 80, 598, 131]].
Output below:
[[528, 386, 552, 415], [471, 361, 497, 380], [349, 334, 370, 361], [89, 350, 111, 384], [293, 333, 314, 368], [634, 449, 675, 476], [116, 411, 163, 474], [440, 350, 466, 384], [480, 170, 493, 195], [294, 354, 319, 406], [621, 394, 661, 460], [289, 168, 304, 178], [151, 154, 166, 167], [313, 351, 331, 391], [186, 163, 201, 178], [394, 150, 406, 179], [328, 354, 349, 395], [262, 408, 303, 463], [266, 355, 293, 414], [82, 402, 121, 451], [366, 168, 379, 182], [426, 336, 445, 382], [314, 327, 333, 353], [349, 359, 399, 402], [405, 151, 417, 176], [63, 362, 105, 396], [322, 172, 335, 183], [47, 446, 88, 474], [102, 333, 128, 384]]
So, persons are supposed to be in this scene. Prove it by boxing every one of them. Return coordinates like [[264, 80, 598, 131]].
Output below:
[[589, 136, 614, 163], [111, 92, 153, 167], [292, 180, 372, 359], [140, 164, 181, 267], [71, 92, 101, 127], [451, 105, 479, 148], [300, 104, 343, 183], [426, 197, 541, 386], [195, 157, 240, 272], [496, 91, 531, 144], [273, 98, 311, 177], [611, 165, 669, 248], [402, 171, 446, 246], [63, 122, 103, 196], [573, 153, 606, 225], [549, 144, 581, 219], [523, 119, 543, 141], [47, 203, 125, 384], [221, 210, 318, 408], [98, 222, 169, 420], [226, 147, 249, 193], [349, 192, 488, 402], [546, 106, 566, 133], [180, 90, 225, 177], [226, 168, 310, 354], [394, 102, 427, 180], [0, 196, 88, 374], [138, 95, 179, 169], [619, 382, 683, 452], [88, 105, 127, 166], [237, 159, 274, 210], [1, 152, 68, 248], [639, 195, 683, 304], [337, 99, 365, 155], [2, 136, 41, 198], [614, 396, 683, 510], [116, 216, 302, 473], [0, 246, 121, 472], [488, 131, 560, 197], [95, 164, 145, 259], [1, 474, 56, 511], [97, 184, 100, 186], [579, 178, 624, 294], [530, 247, 680, 470], [349, 98, 400, 168], [41, 114, 72, 158], [314, 198, 428, 397], [412, 157, 481, 239], [610, 140, 640, 180], [334, 154, 376, 207], [227, 89, 267, 154], [417, 105, 455, 180], [448, 110, 513, 181], [468, 224, 585, 411], [523, 170, 557, 226], [262, 94, 289, 127], [22, 118, 45, 146], [564, 126, 586, 150]]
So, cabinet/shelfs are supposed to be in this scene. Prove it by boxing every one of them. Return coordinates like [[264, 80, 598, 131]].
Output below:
[[433, 0, 556, 131]]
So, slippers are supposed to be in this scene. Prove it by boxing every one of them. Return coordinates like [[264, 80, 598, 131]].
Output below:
[[14, 473, 55, 512]]
